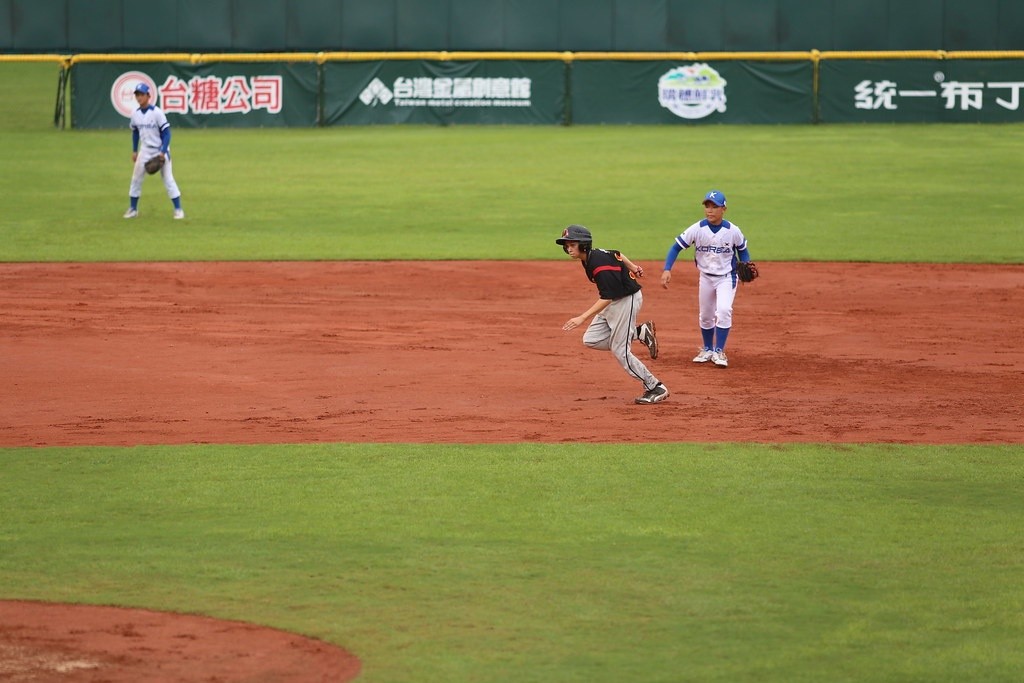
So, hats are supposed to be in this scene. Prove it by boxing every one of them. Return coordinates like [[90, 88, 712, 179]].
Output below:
[[134, 83, 150, 94], [702, 191, 727, 208]]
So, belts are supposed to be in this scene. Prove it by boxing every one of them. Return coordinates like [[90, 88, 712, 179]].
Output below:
[[707, 273, 718, 276]]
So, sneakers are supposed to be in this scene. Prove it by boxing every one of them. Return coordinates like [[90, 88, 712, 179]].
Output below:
[[635, 382, 670, 404], [638, 320, 658, 358], [693, 347, 715, 362], [711, 348, 728, 366]]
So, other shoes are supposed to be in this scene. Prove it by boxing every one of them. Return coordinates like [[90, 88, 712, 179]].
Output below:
[[174, 209, 183, 219], [124, 209, 137, 218]]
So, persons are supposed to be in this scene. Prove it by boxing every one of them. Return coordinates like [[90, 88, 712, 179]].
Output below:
[[123, 83, 185, 219], [660, 190, 757, 367], [556, 224, 670, 406]]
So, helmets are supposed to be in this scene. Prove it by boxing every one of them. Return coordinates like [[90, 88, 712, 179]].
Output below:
[[556, 225, 592, 254]]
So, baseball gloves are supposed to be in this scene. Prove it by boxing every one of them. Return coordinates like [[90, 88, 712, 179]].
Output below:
[[145, 155, 164, 174], [737, 262, 758, 282]]
